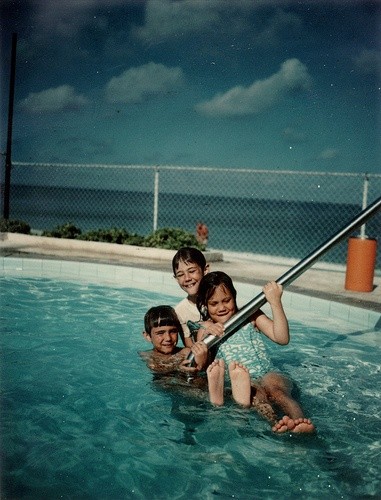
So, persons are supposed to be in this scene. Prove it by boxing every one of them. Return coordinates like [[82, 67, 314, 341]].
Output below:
[[194, 271, 316, 444], [171, 246, 211, 350], [138, 305, 251, 407]]
